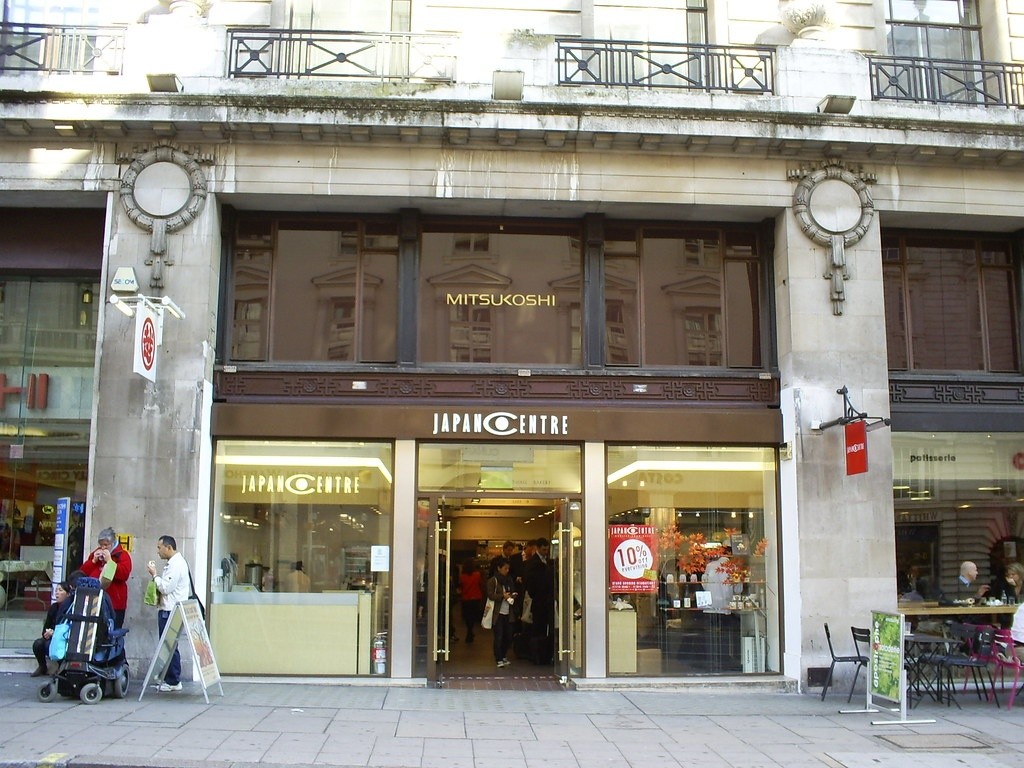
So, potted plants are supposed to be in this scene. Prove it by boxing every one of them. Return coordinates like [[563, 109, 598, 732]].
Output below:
[[781, 4, 831, 40]]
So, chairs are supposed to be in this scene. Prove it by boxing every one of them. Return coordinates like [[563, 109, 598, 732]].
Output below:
[[821, 623, 1024, 711]]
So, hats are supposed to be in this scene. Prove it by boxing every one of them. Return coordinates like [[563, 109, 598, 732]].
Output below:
[[722, 540, 732, 547]]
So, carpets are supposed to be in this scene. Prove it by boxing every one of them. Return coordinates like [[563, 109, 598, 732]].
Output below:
[[24, 591, 52, 610]]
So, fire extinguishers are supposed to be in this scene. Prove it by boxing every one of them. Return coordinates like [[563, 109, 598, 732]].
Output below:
[[372, 632, 389, 673]]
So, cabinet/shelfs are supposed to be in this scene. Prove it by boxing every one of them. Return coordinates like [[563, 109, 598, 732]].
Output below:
[[660, 582, 767, 672]]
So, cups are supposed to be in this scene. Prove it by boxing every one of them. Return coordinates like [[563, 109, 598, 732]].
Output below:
[[904, 622, 911, 634], [981, 597, 986, 606], [1018, 595, 1024, 604], [1008, 596, 1015, 606], [989, 597, 995, 606]]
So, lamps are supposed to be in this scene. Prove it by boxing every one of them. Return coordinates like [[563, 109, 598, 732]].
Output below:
[[109, 294, 185, 320], [819, 385, 891, 432], [815, 94, 856, 114], [491, 69, 525, 100], [146, 73, 184, 92]]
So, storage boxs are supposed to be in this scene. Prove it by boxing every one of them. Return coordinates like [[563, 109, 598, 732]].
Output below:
[[741, 637, 765, 672], [637, 649, 662, 676]]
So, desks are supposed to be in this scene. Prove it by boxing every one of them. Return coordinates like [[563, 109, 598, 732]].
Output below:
[[0, 560, 53, 610], [898, 601, 1019, 623], [906, 633, 963, 710]]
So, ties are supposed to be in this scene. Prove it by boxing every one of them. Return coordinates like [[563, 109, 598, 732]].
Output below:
[[542, 557, 547, 564]]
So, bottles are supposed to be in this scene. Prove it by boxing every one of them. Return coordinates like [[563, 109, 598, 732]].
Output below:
[[1001, 590, 1007, 605], [690, 574, 697, 582], [673, 593, 681, 609], [683, 585, 690, 608], [679, 574, 686, 582], [667, 574, 673, 583], [702, 574, 708, 582]]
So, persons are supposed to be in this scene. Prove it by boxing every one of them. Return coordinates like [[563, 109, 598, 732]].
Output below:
[[147, 535, 192, 691], [940, 560, 1024, 656], [1007, 602, 1024, 647], [897, 563, 928, 600], [261, 561, 276, 591], [30, 528, 132, 678], [423, 549, 484, 643], [701, 546, 743, 671], [284, 560, 311, 592], [0, 507, 85, 601], [486, 537, 554, 668]]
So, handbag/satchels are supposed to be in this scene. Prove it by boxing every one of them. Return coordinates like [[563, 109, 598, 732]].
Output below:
[[481, 598, 494, 630], [49, 624, 70, 662], [189, 594, 206, 620], [144, 575, 159, 606], [99, 560, 117, 590]]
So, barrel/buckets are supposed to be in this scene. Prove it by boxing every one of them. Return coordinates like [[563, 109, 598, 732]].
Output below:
[[245, 563, 262, 589]]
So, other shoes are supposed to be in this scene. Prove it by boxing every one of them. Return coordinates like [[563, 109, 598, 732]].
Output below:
[[497, 658, 511, 667], [450, 634, 459, 641], [30, 666, 48, 677]]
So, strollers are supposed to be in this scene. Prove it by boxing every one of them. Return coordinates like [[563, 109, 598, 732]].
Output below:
[[37, 576, 130, 705]]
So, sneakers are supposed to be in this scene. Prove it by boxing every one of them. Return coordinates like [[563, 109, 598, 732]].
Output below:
[[156, 680, 183, 691], [153, 674, 159, 679]]
